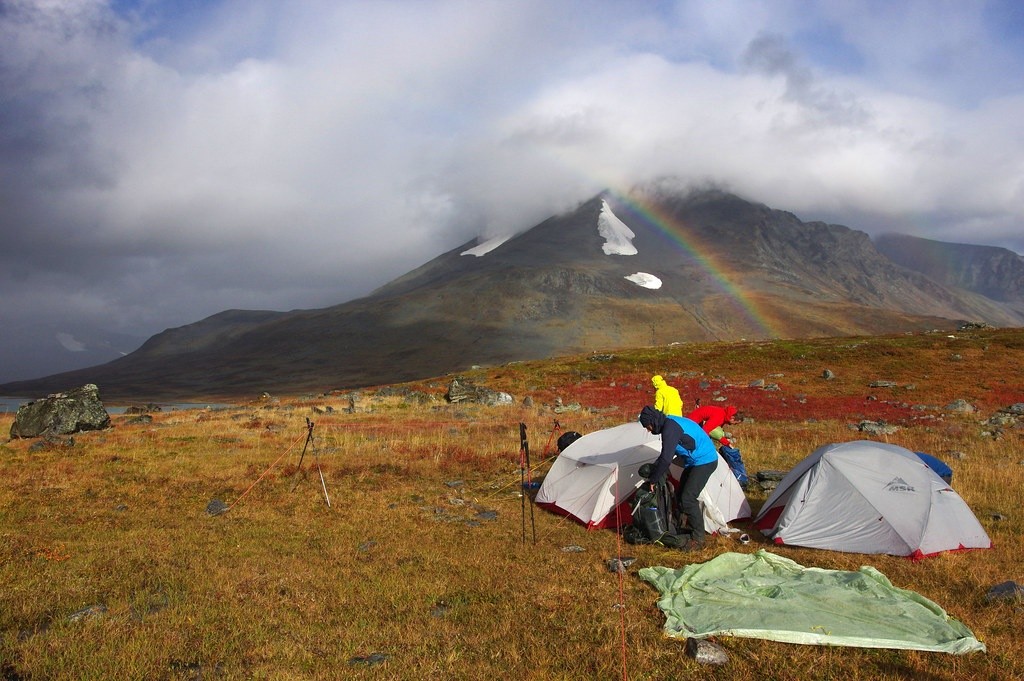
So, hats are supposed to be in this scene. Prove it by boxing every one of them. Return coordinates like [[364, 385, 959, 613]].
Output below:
[[640, 406, 655, 427]]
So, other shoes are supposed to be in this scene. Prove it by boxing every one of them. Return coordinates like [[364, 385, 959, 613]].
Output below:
[[686, 533, 706, 547], [739, 534, 751, 545]]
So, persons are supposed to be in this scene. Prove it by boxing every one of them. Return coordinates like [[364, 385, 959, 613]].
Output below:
[[686, 405, 745, 449], [640, 405, 718, 552], [913, 452, 952, 486], [652, 374, 685, 416]]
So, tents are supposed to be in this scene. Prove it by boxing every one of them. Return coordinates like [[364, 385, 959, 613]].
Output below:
[[535, 422, 752, 531], [754, 440, 995, 561]]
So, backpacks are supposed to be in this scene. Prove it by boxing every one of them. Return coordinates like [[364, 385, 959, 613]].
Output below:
[[718, 446, 748, 481], [631, 481, 673, 541], [558, 431, 582, 451]]
